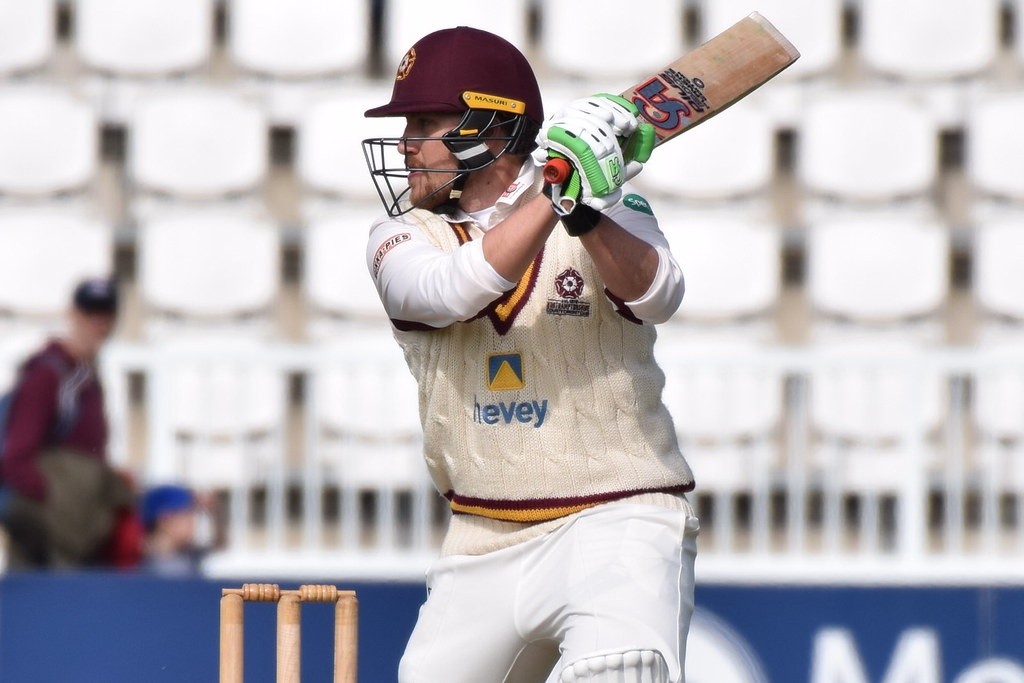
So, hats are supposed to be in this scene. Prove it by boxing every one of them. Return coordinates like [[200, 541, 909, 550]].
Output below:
[[363, 28, 544, 130], [72, 279, 116, 318]]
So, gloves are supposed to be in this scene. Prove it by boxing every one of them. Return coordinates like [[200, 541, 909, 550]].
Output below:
[[534, 93, 655, 217]]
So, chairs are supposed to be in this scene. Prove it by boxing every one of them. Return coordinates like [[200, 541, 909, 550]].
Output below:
[[0, 0, 1023, 504]]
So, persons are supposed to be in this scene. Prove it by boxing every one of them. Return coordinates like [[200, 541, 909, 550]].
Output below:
[[363, 27, 699, 681], [2, 278, 209, 578]]
